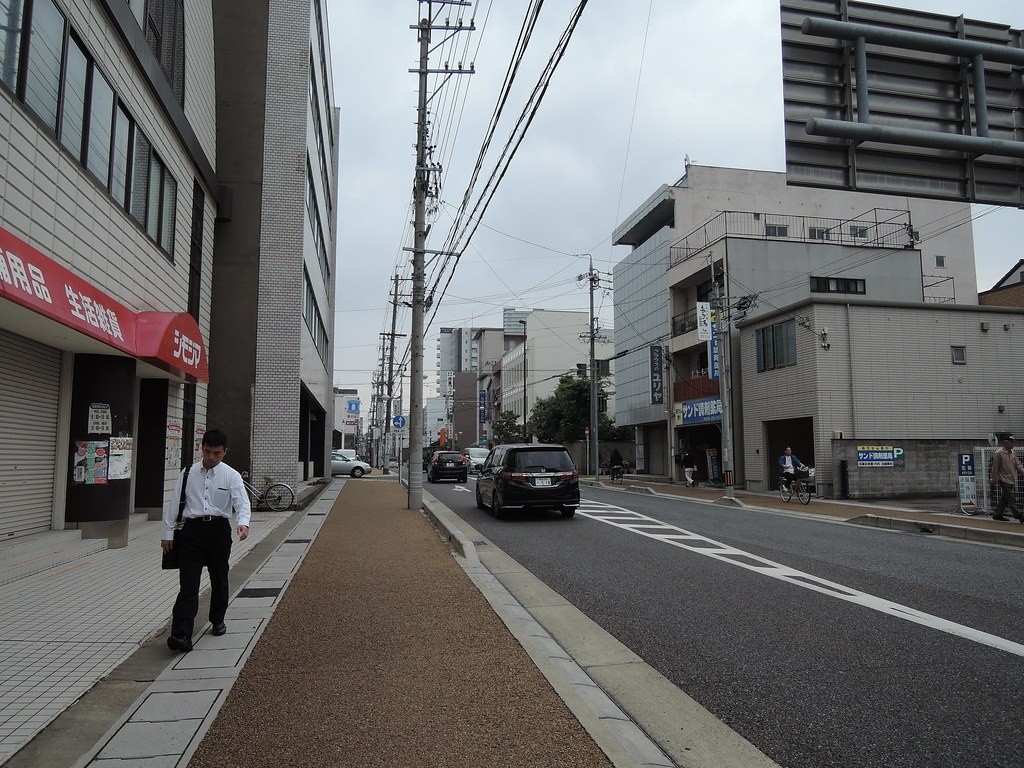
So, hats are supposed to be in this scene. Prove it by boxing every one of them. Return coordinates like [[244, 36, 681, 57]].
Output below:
[[1001, 435, 1014, 440]]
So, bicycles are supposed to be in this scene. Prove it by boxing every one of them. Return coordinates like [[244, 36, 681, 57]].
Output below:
[[609, 460, 623, 485], [242, 469, 294, 512], [779, 466, 811, 505]]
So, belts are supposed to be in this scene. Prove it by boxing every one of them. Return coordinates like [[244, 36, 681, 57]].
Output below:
[[186, 516, 229, 522]]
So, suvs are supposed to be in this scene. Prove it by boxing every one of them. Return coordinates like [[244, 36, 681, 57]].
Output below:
[[427, 451, 467, 483], [464, 447, 491, 475], [476, 443, 580, 520]]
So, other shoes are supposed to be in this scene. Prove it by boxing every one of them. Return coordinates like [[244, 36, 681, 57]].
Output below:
[[686, 483, 688, 487], [692, 481, 696, 487], [611, 477, 613, 481]]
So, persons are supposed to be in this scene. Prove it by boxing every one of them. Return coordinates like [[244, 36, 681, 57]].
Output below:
[[161, 429, 251, 651], [779, 446, 805, 492], [680, 450, 697, 487], [608, 449, 623, 480], [989, 435, 1024, 524]]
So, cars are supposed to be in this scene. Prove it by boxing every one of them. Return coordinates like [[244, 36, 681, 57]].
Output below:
[[388, 457, 398, 468], [331, 452, 372, 478]]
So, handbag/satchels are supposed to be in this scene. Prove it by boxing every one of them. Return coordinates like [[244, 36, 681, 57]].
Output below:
[[694, 465, 698, 471], [161, 529, 179, 569]]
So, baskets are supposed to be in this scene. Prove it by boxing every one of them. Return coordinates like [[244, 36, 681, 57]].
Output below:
[[796, 469, 810, 478]]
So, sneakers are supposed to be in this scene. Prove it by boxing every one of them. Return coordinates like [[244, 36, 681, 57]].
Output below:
[[782, 484, 788, 492]]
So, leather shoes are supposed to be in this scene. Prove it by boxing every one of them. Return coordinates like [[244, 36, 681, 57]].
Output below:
[[1019, 512, 1024, 524], [995, 514, 1009, 521], [167, 635, 193, 653], [212, 622, 226, 636]]
